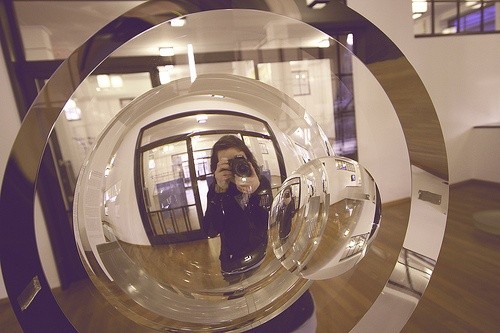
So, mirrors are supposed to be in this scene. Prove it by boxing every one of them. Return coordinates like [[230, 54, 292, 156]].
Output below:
[[1, 1, 500, 333]]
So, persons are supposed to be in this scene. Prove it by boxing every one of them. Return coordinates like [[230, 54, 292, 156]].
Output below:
[[201, 135, 319, 333], [274, 184, 296, 246]]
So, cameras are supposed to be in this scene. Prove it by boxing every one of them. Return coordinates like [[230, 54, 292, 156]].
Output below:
[[226, 154, 251, 178], [284, 193, 290, 198]]
